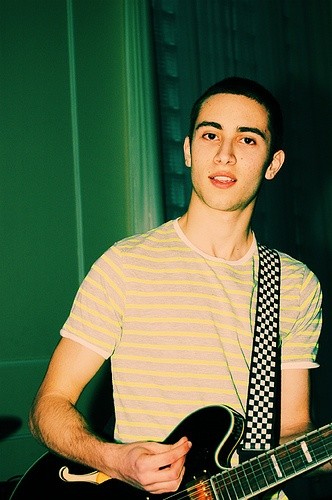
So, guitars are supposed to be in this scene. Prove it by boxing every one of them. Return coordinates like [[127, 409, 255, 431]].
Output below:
[[7, 403, 331, 500]]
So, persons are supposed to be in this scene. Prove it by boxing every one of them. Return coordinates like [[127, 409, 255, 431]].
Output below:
[[28, 75, 332, 500]]
